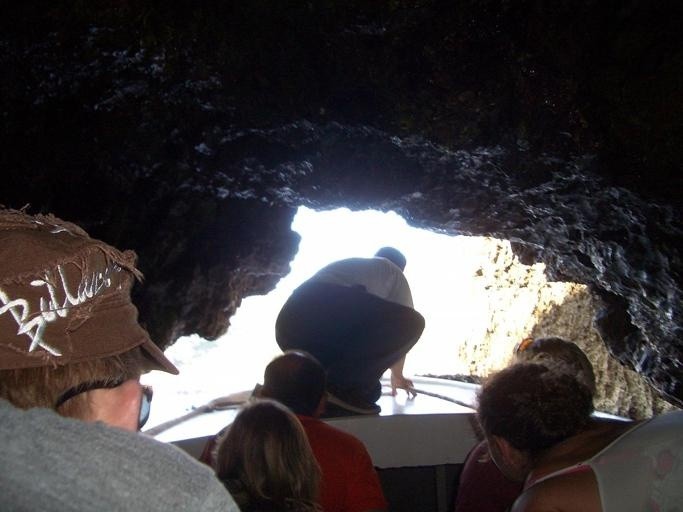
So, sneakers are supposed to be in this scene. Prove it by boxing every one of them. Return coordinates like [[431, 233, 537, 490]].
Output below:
[[325, 385, 381, 414]]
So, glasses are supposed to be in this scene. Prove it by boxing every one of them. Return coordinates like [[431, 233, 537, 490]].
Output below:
[[56, 373, 151, 431]]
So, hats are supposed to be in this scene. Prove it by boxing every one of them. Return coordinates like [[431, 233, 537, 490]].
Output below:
[[0, 207, 179, 375]]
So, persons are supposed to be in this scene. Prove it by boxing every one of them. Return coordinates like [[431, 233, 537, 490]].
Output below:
[[273, 246, 425, 414], [454, 334, 632, 512], [472, 362, 682, 512], [199, 348, 390, 511], [211, 396, 323, 511], [0, 200, 241, 512]]
